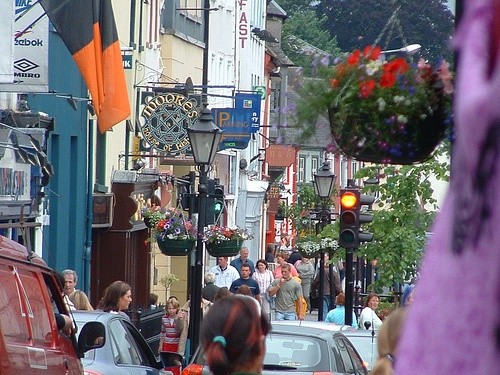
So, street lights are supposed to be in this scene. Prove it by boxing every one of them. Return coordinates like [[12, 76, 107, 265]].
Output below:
[[312, 157, 339, 322], [183, 102, 224, 366], [361, 171, 381, 293]]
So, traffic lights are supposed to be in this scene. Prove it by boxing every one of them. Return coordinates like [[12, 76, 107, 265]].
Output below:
[[358, 193, 375, 242], [338, 188, 361, 250], [213, 185, 224, 214]]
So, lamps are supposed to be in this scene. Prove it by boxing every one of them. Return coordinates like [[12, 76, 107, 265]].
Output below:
[[96, 183, 109, 193], [0, 122, 54, 186], [132, 158, 146, 171], [280, 180, 293, 195], [15, 90, 96, 116], [251, 26, 280, 45]]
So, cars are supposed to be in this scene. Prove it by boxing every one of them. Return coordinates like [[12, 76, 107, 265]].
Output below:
[[72, 310, 183, 374], [262, 319, 378, 375], [0, 233, 85, 375]]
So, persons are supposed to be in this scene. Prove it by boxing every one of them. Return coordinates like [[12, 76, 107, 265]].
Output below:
[[311, 251, 344, 321], [229, 264, 260, 301], [150, 291, 214, 367], [273, 252, 299, 279], [325, 293, 359, 330], [236, 284, 252, 296], [202, 272, 220, 303], [359, 292, 383, 328], [54, 312, 72, 337], [200, 294, 272, 375], [268, 264, 304, 320], [209, 256, 241, 290], [61, 269, 94, 311], [215, 287, 233, 302], [265, 243, 316, 313], [370, 307, 409, 375], [252, 259, 275, 321], [93, 336, 104, 347], [230, 246, 255, 277], [94, 280, 133, 321]]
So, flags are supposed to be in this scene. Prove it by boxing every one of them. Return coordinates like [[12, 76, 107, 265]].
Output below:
[[38, 0, 131, 135]]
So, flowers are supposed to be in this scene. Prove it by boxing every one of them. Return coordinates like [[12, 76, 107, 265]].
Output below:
[[140, 204, 198, 245], [199, 223, 254, 249], [295, 44, 456, 162]]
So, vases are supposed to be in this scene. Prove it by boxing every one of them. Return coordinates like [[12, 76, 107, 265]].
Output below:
[[206, 239, 244, 256], [327, 108, 448, 169], [158, 239, 196, 256]]
